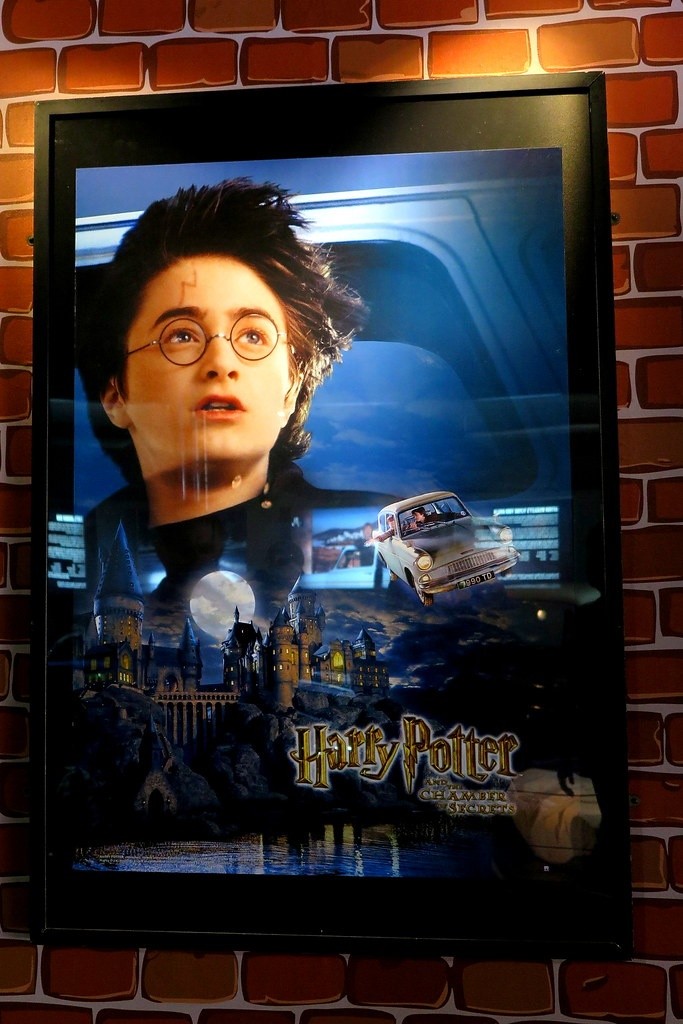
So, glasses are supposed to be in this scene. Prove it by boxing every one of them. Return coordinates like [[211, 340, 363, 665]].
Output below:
[[124, 312, 291, 366]]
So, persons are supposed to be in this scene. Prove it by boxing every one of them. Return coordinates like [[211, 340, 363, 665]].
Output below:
[[365, 507, 443, 547], [81, 175, 370, 659]]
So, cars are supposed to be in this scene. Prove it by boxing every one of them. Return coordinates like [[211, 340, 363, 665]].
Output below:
[[371, 487, 522, 607], [48, 170, 572, 616]]
